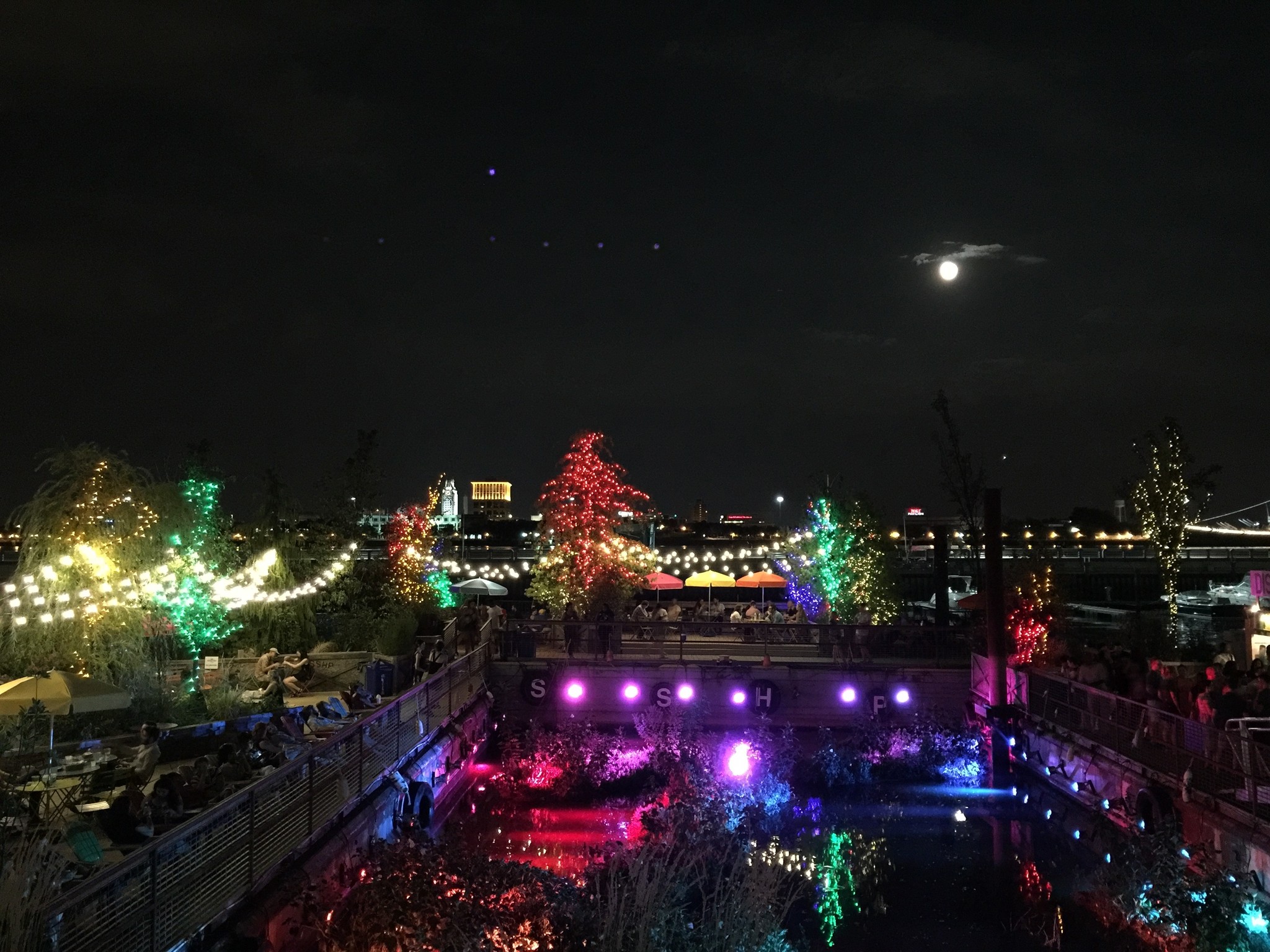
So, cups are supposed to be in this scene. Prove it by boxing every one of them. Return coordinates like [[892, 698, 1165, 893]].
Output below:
[[89, 757, 96, 767], [103, 747, 111, 758], [31, 775, 39, 785], [65, 756, 72, 765], [376, 694, 381, 704]]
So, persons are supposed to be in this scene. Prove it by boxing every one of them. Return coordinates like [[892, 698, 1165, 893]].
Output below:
[[596, 603, 615, 659], [0, 771, 46, 827], [1060, 646, 1270, 778], [561, 602, 580, 657], [104, 680, 379, 843], [282, 648, 310, 698], [828, 611, 843, 663], [869, 612, 988, 658], [629, 598, 830, 643], [855, 604, 874, 664], [458, 599, 551, 660], [90, 722, 160, 792], [255, 648, 281, 695], [415, 637, 448, 717]]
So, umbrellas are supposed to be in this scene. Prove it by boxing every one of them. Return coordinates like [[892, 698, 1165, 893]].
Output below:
[[0, 667, 131, 824], [451, 577, 508, 607], [736, 571, 787, 613], [685, 569, 735, 632], [957, 590, 1008, 610], [640, 572, 683, 602]]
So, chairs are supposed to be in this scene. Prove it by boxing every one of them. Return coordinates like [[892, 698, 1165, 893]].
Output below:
[[0, 662, 397, 896], [624, 607, 811, 642]]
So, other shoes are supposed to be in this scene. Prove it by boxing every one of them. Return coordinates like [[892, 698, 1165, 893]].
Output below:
[[28, 818, 45, 826]]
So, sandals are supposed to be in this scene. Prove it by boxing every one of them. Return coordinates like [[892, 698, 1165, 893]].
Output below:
[[298, 688, 303, 697], [290, 692, 298, 698]]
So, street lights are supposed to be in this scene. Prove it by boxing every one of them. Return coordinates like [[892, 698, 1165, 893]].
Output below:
[[776, 496, 784, 522]]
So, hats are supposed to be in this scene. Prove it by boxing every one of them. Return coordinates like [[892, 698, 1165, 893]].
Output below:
[[269, 647, 280, 654]]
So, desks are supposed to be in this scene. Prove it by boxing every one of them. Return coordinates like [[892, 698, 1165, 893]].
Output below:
[[134, 722, 178, 757], [700, 614, 717, 636], [746, 619, 771, 640], [15, 779, 81, 826], [271, 666, 298, 694], [55, 754, 118, 794], [39, 764, 99, 808]]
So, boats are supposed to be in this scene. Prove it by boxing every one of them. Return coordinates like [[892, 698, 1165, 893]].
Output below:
[[908, 575, 978, 620], [1063, 602, 1137, 631], [1159, 571, 1270, 631]]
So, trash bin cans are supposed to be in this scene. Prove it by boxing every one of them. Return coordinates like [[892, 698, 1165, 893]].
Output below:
[[517, 625, 538, 657], [498, 631, 517, 657], [366, 660, 394, 697]]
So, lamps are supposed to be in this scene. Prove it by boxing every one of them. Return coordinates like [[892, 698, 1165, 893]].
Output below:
[[1011, 733, 1262, 890], [432, 713, 508, 786]]
